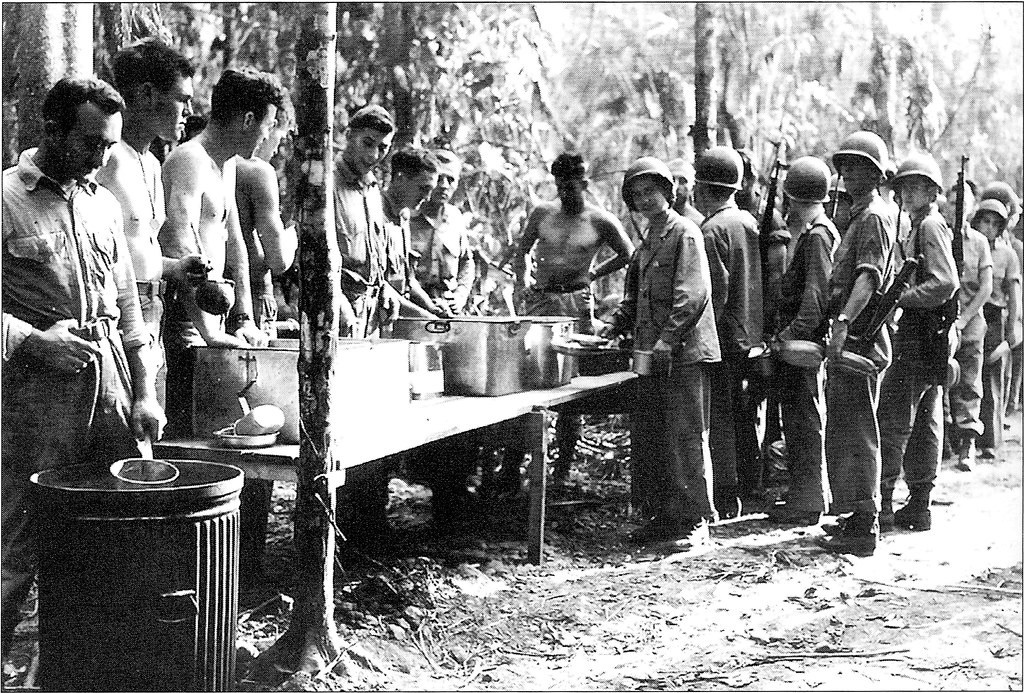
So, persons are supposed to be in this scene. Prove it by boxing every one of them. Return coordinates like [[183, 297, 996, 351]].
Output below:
[[1, 37, 475, 653], [594, 132, 1023, 556], [481, 151, 632, 494]]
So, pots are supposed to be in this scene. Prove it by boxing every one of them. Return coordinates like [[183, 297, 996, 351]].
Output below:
[[192, 340, 417, 435], [394, 316, 580, 396]]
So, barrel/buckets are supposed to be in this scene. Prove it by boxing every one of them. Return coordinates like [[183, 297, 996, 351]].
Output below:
[[29, 461, 247, 692]]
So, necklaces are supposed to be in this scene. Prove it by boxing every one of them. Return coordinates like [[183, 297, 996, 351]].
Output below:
[[138, 153, 159, 230]]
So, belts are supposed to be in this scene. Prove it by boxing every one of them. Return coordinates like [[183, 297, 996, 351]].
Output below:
[[69, 321, 108, 341], [529, 282, 585, 294]]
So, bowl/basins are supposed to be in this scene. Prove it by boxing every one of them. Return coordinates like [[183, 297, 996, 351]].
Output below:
[[781, 340, 825, 368], [828, 351, 877, 376], [631, 350, 674, 378], [945, 359, 961, 390], [213, 430, 280, 447]]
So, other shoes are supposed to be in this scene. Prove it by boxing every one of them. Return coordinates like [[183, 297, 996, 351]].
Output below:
[[619, 436, 994, 556]]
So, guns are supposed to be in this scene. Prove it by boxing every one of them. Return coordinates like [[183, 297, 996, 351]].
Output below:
[[761, 159, 781, 273], [829, 173, 842, 224], [862, 257, 919, 344], [927, 153, 970, 386]]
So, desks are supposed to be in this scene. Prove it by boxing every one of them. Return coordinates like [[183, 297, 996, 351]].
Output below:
[[152, 346, 767, 566]]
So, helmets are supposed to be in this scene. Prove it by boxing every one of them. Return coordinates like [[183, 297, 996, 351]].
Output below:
[[784, 156, 831, 204], [971, 199, 1009, 237], [694, 146, 745, 190], [890, 154, 943, 195], [832, 131, 888, 184], [621, 157, 675, 212], [981, 182, 1016, 216]]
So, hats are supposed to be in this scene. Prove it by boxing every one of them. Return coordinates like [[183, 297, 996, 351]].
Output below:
[[348, 105, 395, 135]]
